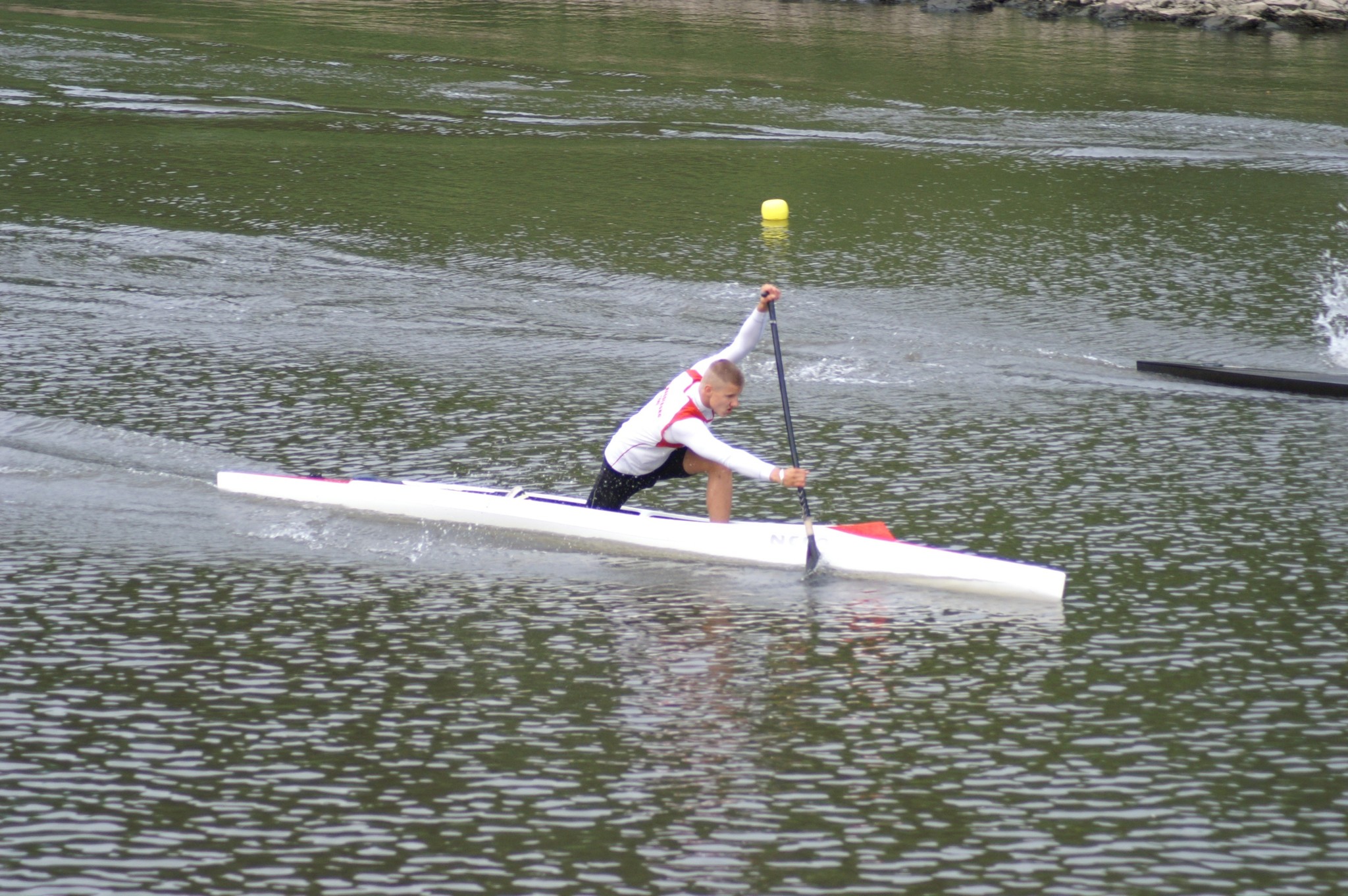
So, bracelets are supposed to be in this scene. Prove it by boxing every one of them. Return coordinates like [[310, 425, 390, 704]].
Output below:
[[779, 469, 784, 486]]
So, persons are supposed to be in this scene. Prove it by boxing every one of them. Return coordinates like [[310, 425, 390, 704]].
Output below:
[[586, 284, 809, 523]]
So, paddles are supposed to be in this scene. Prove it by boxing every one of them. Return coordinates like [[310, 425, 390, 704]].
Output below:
[[760, 292, 823, 574]]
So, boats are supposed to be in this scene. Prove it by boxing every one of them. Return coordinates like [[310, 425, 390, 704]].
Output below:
[[217, 471, 1066, 602], [1136, 359, 1348, 395]]
